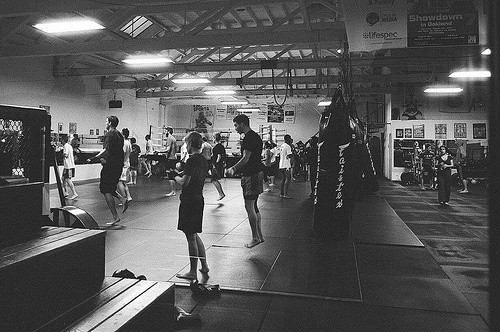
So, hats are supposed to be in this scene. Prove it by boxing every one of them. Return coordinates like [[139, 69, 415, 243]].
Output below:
[[165, 126, 173, 135]]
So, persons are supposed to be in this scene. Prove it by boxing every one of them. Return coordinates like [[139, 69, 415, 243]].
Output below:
[[170, 132, 209, 279], [200, 137, 213, 176], [279, 134, 292, 199], [459, 153, 468, 194], [436, 146, 454, 205], [180, 137, 189, 164], [71, 134, 80, 143], [143, 135, 155, 178], [420, 144, 435, 190], [117, 128, 132, 205], [60, 135, 79, 199], [211, 132, 226, 200], [225, 114, 266, 248], [164, 127, 177, 196], [87, 116, 128, 225], [126, 137, 140, 185], [261, 142, 276, 192], [307, 137, 318, 196]]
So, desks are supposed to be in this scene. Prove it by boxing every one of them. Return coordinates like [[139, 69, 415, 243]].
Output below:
[[225, 155, 242, 177]]
